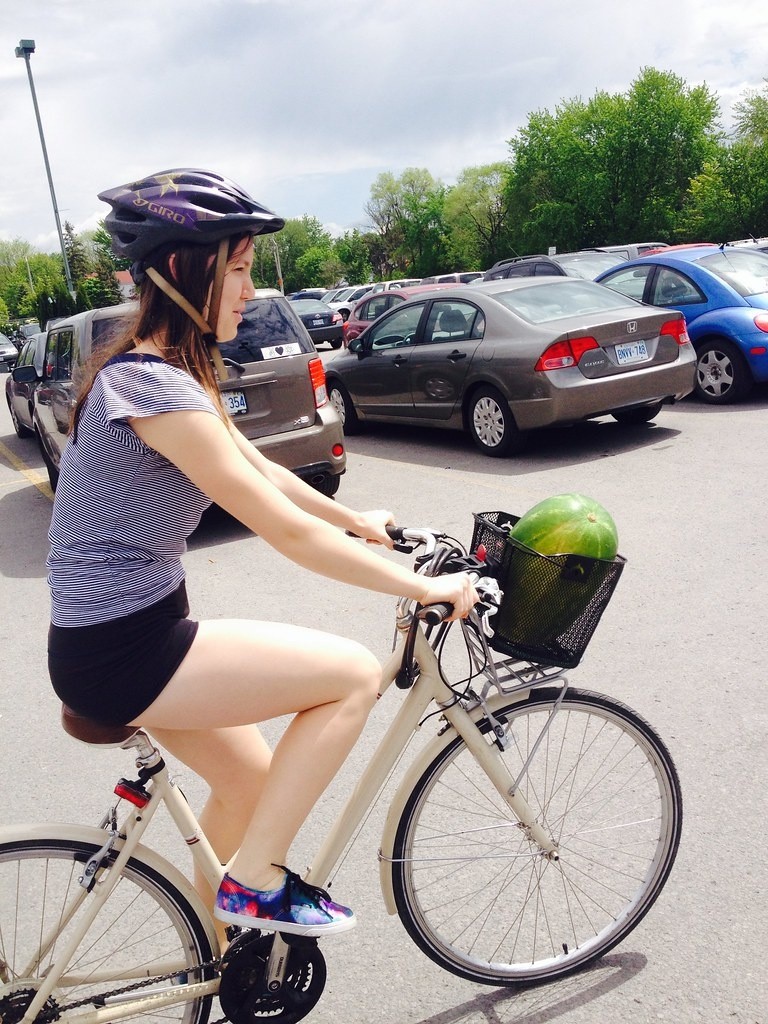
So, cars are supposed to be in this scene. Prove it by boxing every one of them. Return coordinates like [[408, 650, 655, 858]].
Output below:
[[325, 276, 699, 458], [5, 329, 47, 439], [343, 282, 485, 340], [287, 299, 344, 350], [286, 271, 502, 319], [15, 323, 42, 352], [596, 239, 768, 273], [0, 332, 19, 372], [9, 331, 17, 344], [594, 245, 768, 404], [485, 249, 648, 303], [45, 315, 71, 332]]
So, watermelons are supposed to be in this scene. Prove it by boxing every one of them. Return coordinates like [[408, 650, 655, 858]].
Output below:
[[498, 494, 619, 646]]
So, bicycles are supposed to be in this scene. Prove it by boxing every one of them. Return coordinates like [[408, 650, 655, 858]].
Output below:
[[1, 511, 685, 1024]]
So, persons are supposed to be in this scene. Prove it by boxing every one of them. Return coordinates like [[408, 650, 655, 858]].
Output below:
[[45, 168, 481, 989]]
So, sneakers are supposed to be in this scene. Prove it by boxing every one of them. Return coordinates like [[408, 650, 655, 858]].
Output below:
[[172, 970, 220, 996], [214, 863, 357, 937]]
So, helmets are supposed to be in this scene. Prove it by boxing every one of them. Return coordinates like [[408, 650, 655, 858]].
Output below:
[[97, 169, 285, 286]]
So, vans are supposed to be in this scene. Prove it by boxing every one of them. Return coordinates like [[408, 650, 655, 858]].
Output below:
[[13, 287, 345, 500]]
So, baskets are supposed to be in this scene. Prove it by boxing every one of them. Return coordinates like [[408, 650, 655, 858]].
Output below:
[[464, 511, 627, 669]]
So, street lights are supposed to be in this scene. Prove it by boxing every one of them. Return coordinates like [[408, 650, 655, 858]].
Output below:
[[15, 38, 74, 294]]
